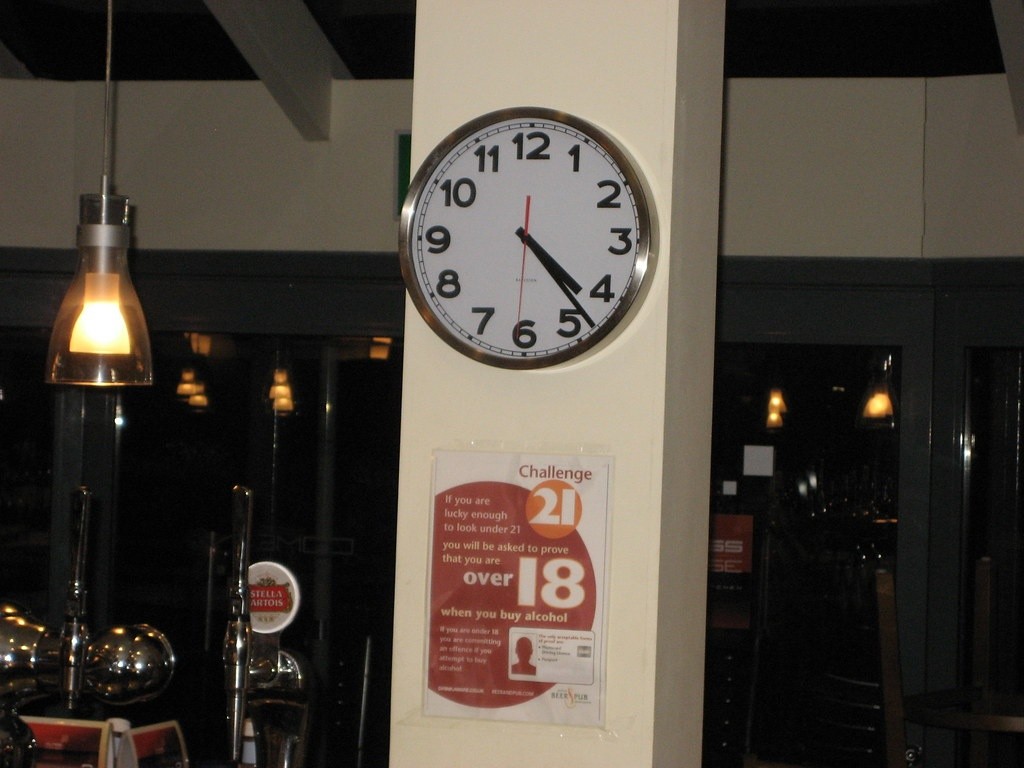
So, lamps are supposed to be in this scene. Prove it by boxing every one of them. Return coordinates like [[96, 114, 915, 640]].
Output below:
[[43, 0, 154, 388]]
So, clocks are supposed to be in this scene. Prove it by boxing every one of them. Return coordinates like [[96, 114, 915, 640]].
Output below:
[[399, 106, 659, 372]]
